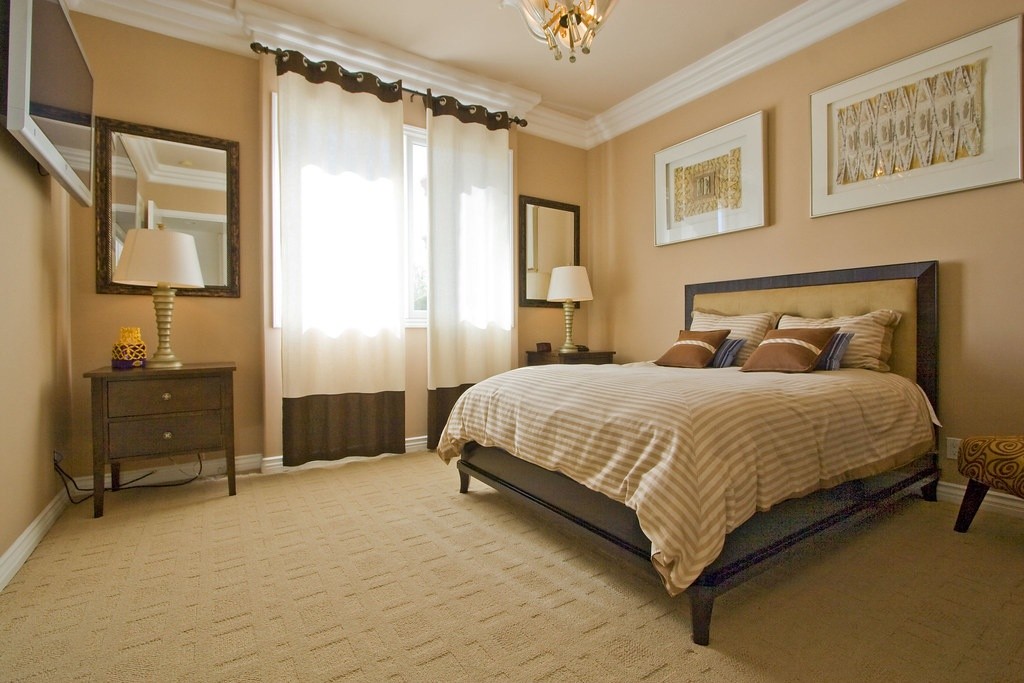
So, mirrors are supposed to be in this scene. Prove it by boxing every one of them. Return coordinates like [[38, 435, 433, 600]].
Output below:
[[518, 194, 581, 310], [94, 116, 242, 299]]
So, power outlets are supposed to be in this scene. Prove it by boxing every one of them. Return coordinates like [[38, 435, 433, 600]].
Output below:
[[946, 437, 962, 459]]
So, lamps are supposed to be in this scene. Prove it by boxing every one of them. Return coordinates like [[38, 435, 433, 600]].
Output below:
[[500, 0, 618, 63], [547, 266, 593, 353], [113, 224, 205, 368]]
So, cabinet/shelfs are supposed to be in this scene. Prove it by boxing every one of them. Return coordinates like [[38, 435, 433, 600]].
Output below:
[[527, 351, 617, 366], [83, 362, 237, 518]]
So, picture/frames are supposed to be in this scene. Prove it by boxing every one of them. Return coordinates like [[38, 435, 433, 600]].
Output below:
[[808, 13, 1024, 219], [652, 109, 768, 247], [526, 204, 537, 274]]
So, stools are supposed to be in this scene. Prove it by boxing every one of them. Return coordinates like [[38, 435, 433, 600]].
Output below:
[[954, 435, 1024, 533]]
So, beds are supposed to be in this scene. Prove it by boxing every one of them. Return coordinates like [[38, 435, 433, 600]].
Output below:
[[436, 260, 942, 647]]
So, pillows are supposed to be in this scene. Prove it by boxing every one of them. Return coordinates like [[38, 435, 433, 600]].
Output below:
[[690, 311, 785, 366], [738, 327, 841, 374], [712, 338, 747, 368], [817, 332, 855, 370], [652, 330, 731, 368], [778, 309, 903, 372]]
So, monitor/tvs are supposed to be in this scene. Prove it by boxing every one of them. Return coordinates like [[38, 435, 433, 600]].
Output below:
[[6, 0, 96, 207]]
